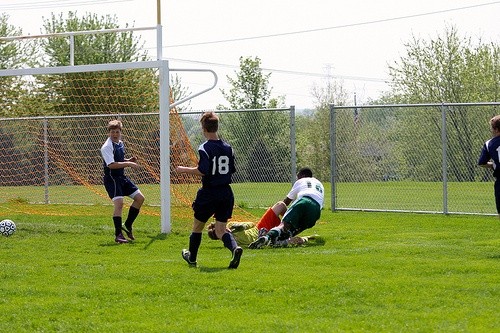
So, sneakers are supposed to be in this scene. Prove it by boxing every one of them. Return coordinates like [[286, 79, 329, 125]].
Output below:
[[248, 236, 268, 249], [182, 249, 196, 267], [115, 233, 129, 242], [122, 222, 135, 240], [228, 247, 243, 269], [308, 235, 322, 241]]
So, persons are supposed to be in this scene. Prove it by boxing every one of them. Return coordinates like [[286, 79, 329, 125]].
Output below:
[[177, 110, 243, 269], [247, 166, 325, 249], [100, 120, 145, 243], [207, 201, 326, 249], [478, 115, 500, 216]]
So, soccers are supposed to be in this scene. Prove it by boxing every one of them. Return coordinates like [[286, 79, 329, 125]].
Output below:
[[0, 219, 16, 237]]
